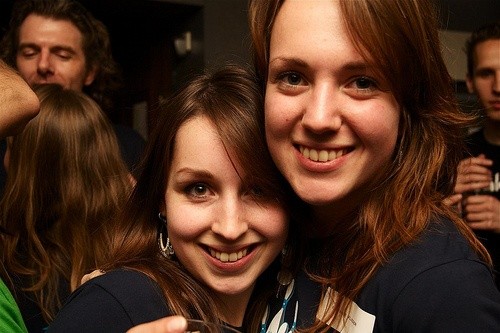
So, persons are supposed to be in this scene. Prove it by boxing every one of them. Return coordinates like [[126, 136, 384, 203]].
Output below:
[[250, 1, 500, 333], [39, 64, 290, 333], [0, 0, 179, 333], [437, 23, 500, 287]]
